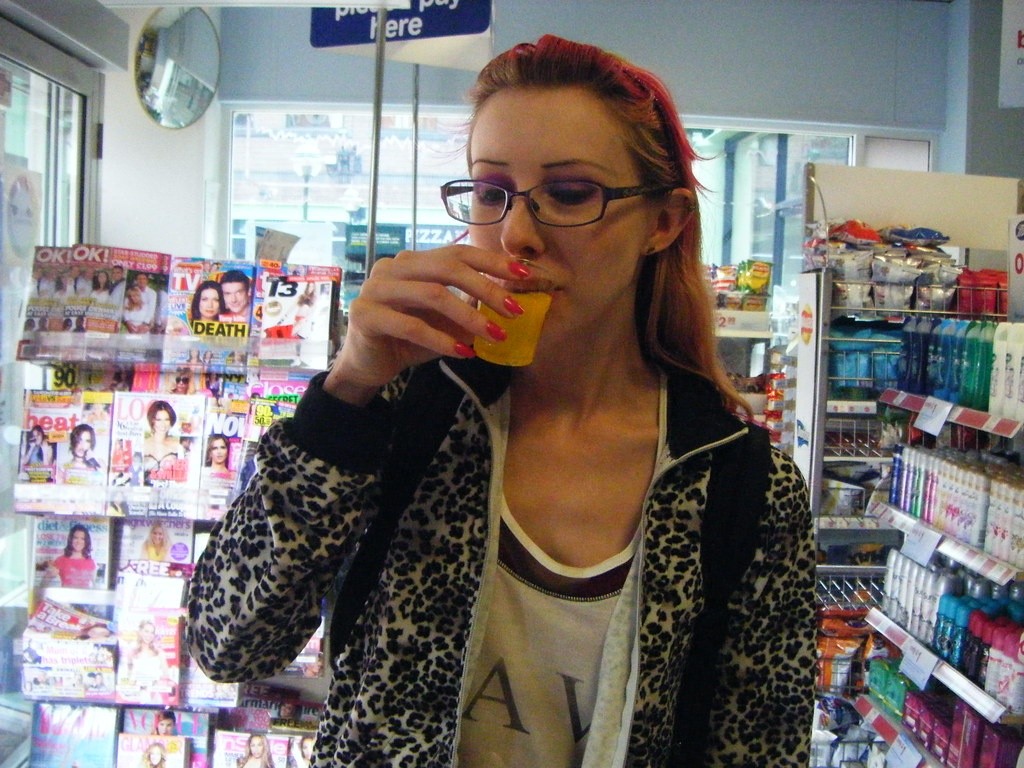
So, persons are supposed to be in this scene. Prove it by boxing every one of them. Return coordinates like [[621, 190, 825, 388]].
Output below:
[[189, 31, 815, 768]]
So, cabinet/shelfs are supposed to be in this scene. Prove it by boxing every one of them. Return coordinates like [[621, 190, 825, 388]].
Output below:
[[711, 307, 774, 436], [855, 388, 1024, 768], [799, 266, 1008, 768], [10, 327, 330, 768]]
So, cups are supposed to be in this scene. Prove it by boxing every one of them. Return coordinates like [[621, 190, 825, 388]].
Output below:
[[474, 257, 561, 369]]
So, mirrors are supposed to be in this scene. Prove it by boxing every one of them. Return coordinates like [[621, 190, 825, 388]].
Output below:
[[132, 6, 221, 129]]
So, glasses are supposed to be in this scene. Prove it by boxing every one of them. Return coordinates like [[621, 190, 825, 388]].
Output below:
[[439, 178, 664, 228]]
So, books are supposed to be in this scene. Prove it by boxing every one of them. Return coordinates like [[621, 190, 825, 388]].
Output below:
[[10, 234, 1024, 768]]
